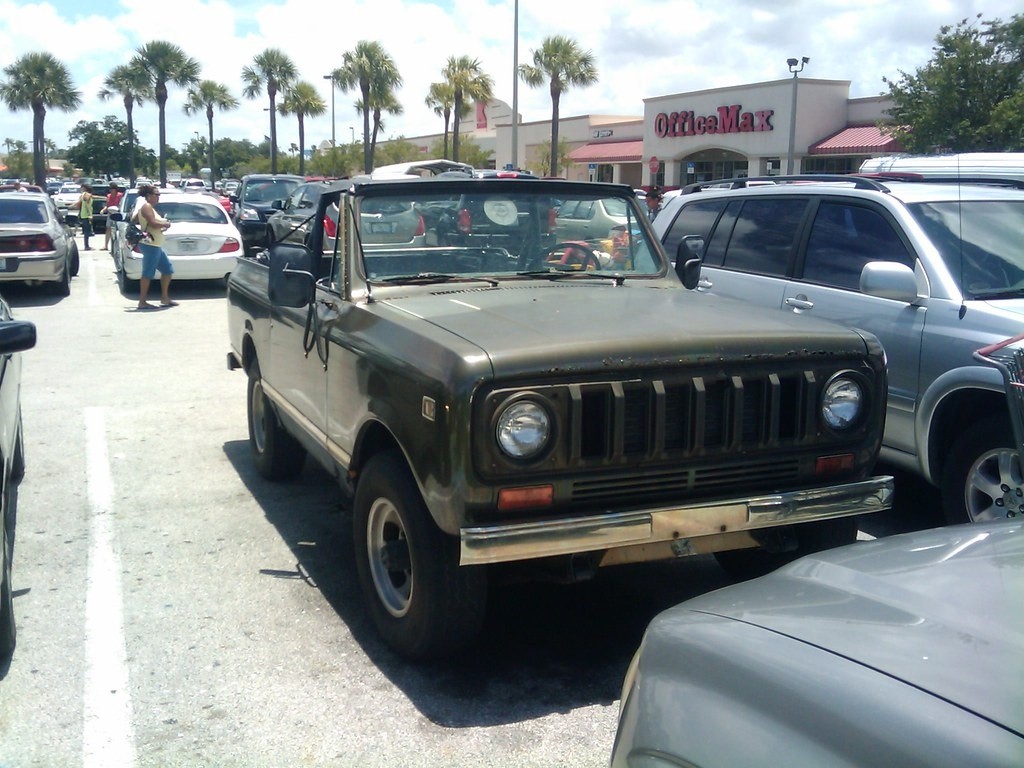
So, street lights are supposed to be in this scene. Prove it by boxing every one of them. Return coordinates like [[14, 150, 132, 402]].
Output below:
[[349, 127, 354, 144], [323, 75, 335, 177], [264, 108, 272, 160], [194, 132, 199, 140], [787, 57, 810, 183]]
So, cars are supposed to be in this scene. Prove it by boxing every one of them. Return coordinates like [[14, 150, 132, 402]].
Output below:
[[859, 152, 1024, 189], [661, 181, 776, 210], [556, 189, 650, 249], [197, 192, 231, 212], [0, 177, 242, 207], [0, 192, 79, 296], [610, 518, 1024, 768], [109, 194, 245, 292], [0, 292, 37, 649]]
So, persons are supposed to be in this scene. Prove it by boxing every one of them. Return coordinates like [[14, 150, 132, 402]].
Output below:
[[14, 182, 28, 192], [68, 184, 94, 250], [136, 184, 179, 309], [99, 182, 123, 250], [646, 191, 664, 222]]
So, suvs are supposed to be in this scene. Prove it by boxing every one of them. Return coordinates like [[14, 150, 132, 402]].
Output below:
[[226, 177, 896, 665], [230, 174, 306, 256], [413, 165, 557, 273], [632, 171, 1024, 523], [265, 177, 426, 281]]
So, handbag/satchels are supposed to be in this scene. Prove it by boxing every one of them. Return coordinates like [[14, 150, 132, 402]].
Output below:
[[124, 225, 146, 245]]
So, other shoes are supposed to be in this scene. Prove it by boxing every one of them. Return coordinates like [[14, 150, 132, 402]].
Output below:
[[85, 246, 91, 250], [100, 248, 108, 250]]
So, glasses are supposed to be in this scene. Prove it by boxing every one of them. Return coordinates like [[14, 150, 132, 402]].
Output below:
[[152, 193, 160, 196]]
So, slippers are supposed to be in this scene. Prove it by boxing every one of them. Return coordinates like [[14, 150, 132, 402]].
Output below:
[[137, 303, 159, 309], [159, 299, 179, 307]]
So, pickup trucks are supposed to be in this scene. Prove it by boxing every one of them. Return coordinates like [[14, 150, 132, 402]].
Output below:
[[82, 185, 129, 231]]
